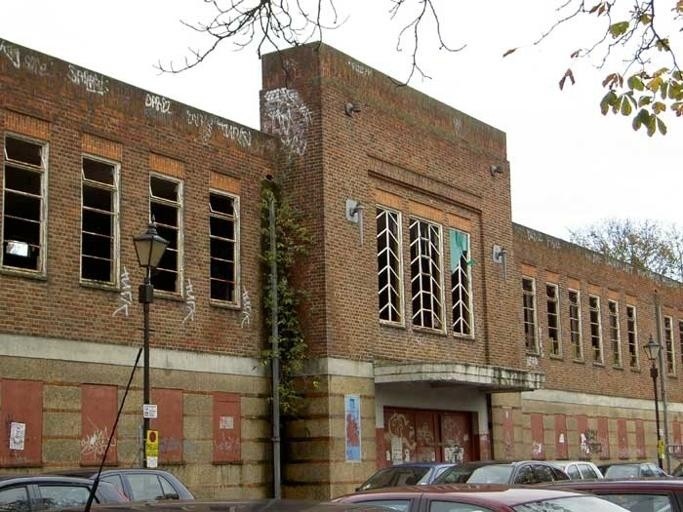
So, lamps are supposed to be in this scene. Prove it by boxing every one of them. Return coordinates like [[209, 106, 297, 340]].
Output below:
[[496, 247, 508, 286], [350, 201, 365, 249]]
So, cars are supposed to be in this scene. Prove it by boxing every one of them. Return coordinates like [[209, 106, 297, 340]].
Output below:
[[58, 468, 193, 504], [547, 460, 604, 479], [39, 498, 404, 511], [598, 461, 676, 479], [672, 463, 683, 477], [535, 479, 682, 512], [425, 461, 572, 484], [354, 462, 458, 491], [322, 483, 632, 512], [0, 473, 132, 512]]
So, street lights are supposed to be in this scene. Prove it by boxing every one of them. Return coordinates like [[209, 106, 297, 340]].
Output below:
[[133, 215, 169, 467], [642, 333, 665, 470]]
